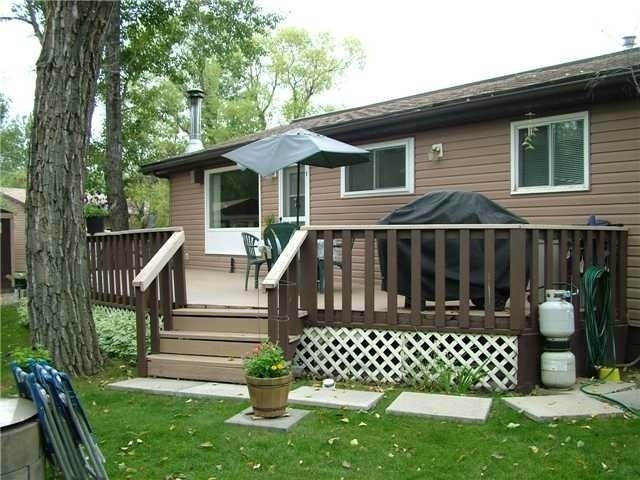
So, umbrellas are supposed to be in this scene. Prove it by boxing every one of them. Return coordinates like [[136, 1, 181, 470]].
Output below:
[[221, 122, 373, 229]]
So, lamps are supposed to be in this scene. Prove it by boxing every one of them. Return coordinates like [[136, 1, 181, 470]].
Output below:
[[428, 143, 443, 161]]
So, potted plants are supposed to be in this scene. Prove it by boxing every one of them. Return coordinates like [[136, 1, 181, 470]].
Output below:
[[245, 374, 290, 419], [83, 201, 112, 233]]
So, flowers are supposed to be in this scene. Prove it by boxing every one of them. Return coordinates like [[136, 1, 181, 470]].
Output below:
[[242, 340, 290, 378]]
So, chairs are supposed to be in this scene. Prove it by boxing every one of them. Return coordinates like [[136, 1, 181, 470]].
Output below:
[[242, 221, 342, 294], [10, 358, 110, 480]]
